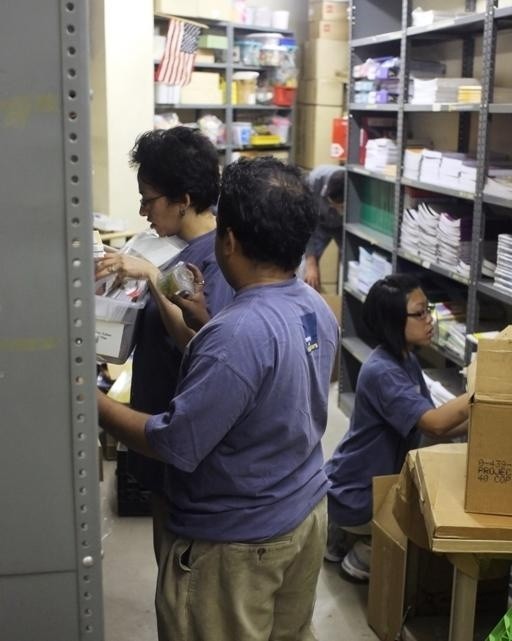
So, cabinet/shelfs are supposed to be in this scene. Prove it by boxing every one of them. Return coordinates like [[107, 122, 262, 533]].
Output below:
[[153, 10, 297, 195], [339, 1, 403, 420], [396, 1, 487, 410], [468, 1, 511, 357]]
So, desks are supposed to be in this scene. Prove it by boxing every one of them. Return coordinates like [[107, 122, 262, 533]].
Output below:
[[408, 442, 512, 641]]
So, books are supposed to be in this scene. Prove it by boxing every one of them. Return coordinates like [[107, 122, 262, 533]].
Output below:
[[411, 5, 471, 24], [352, 55, 512, 103], [358, 178, 512, 295], [357, 115, 512, 201], [91, 209, 190, 407], [345, 246, 512, 409]]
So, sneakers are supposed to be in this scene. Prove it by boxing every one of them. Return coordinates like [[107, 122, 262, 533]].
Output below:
[[324, 543, 371, 584]]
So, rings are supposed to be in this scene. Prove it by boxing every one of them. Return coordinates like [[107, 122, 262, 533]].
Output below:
[[195, 282, 207, 287]]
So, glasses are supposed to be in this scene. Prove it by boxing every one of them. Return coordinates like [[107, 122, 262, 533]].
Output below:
[[140, 191, 168, 209], [406, 303, 436, 320]]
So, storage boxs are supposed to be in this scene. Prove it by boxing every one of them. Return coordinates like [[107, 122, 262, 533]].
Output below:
[[370, 452, 463, 641], [461, 323, 512, 519], [298, 0, 351, 330], [95, 278, 152, 365]]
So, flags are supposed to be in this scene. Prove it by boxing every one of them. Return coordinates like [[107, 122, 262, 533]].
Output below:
[[154, 18, 203, 86]]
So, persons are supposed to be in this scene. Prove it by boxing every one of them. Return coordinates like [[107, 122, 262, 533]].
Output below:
[[96, 150, 346, 641], [298, 162, 346, 297], [96, 125, 234, 520], [323, 269, 512, 584]]
